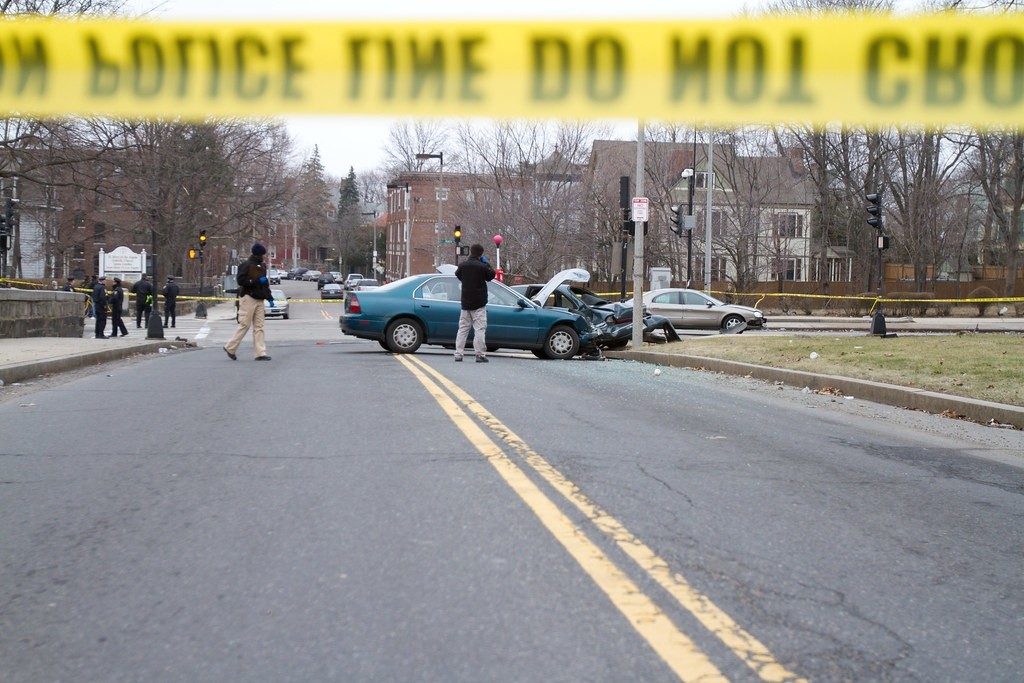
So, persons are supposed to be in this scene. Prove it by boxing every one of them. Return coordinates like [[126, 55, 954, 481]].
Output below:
[[162, 275, 179, 328], [63, 277, 75, 292], [223, 243, 274, 359], [94, 276, 109, 338], [131, 273, 153, 328], [146, 274, 152, 313], [89, 276, 98, 319], [82, 274, 89, 315], [108, 277, 127, 337], [454, 244, 495, 363]]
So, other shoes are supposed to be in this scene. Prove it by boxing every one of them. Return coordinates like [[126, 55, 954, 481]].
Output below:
[[171, 325, 175, 327], [145, 326, 147, 328], [108, 334, 117, 337], [255, 355, 271, 360], [96, 335, 109, 339], [163, 325, 168, 328], [120, 332, 128, 336], [137, 325, 142, 328], [455, 357, 462, 361], [476, 355, 488, 362], [223, 346, 237, 360]]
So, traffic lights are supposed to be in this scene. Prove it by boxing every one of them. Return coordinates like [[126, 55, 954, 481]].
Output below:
[[189, 249, 196, 260], [877, 236, 890, 251], [454, 224, 462, 242], [866, 192, 883, 229], [198, 229, 207, 247], [669, 204, 690, 238]]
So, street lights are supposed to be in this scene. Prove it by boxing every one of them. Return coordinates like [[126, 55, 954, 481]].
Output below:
[[415, 151, 444, 267], [493, 234, 504, 283], [361, 210, 376, 280]]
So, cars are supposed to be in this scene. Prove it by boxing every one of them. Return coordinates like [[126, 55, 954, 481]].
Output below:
[[275, 267, 344, 283], [338, 271, 592, 360], [350, 279, 379, 291], [435, 263, 682, 362], [320, 283, 344, 300], [263, 289, 292, 319], [343, 273, 365, 290], [267, 270, 281, 285], [625, 287, 765, 335]]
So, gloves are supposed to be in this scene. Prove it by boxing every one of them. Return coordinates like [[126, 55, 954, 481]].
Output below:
[[269, 301, 274, 307], [259, 276, 267, 284], [480, 256, 487, 264]]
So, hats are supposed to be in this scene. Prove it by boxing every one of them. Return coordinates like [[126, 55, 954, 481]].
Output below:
[[168, 275, 174, 279], [252, 243, 266, 255], [114, 278, 121, 282], [67, 277, 74, 280], [99, 276, 106, 280]]
